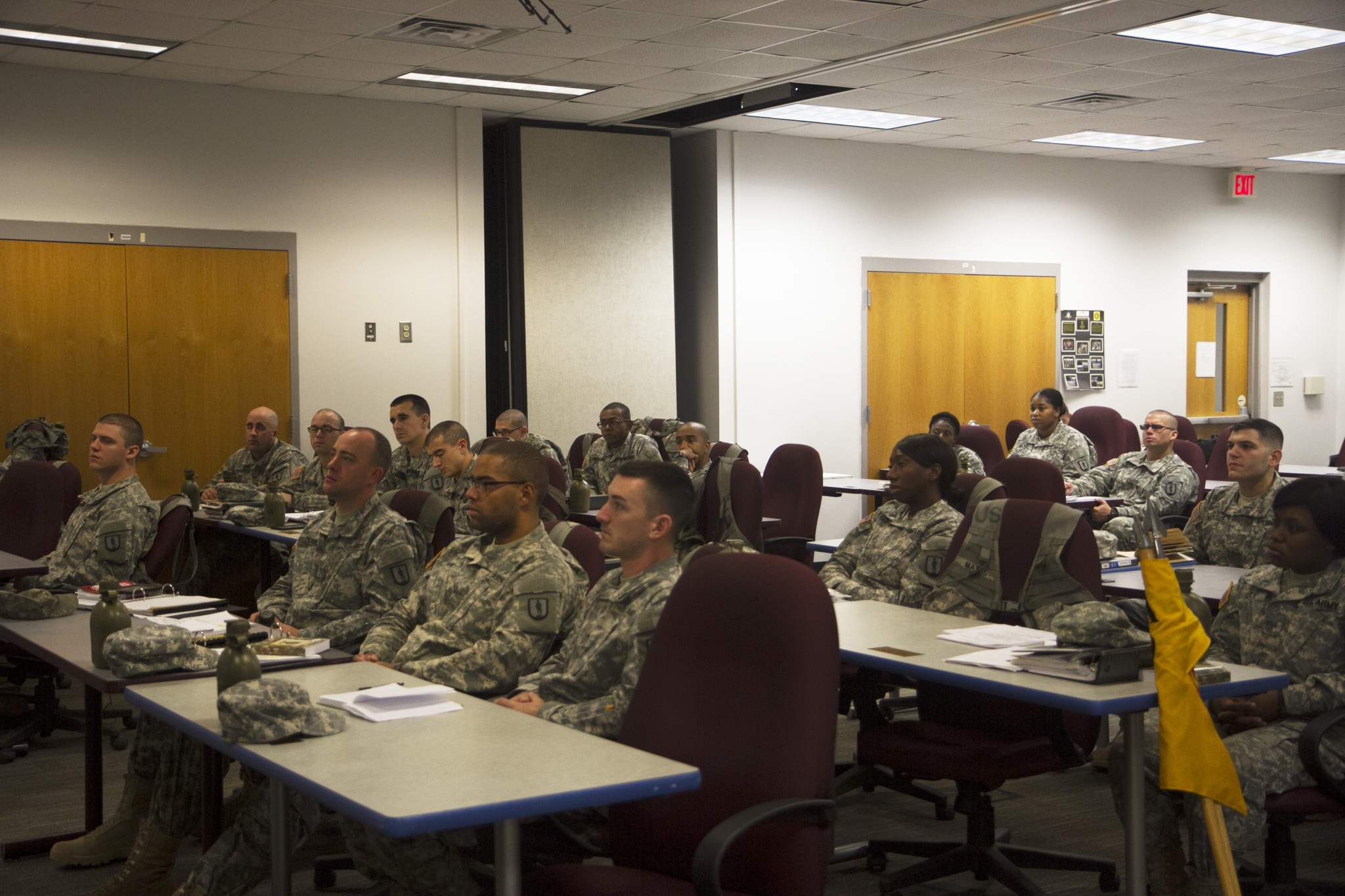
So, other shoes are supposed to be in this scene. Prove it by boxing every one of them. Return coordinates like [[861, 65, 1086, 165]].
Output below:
[[1090, 729, 1124, 771]]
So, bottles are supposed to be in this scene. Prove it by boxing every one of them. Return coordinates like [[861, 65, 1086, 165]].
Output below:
[[217, 619, 261, 737], [181, 468, 200, 512], [958, 458, 974, 474], [90, 576, 132, 669], [569, 468, 590, 513], [1174, 568, 1211, 662], [726, 539, 744, 552], [264, 480, 285, 527]]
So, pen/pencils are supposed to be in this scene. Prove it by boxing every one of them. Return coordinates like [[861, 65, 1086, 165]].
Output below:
[[353, 681, 404, 691]]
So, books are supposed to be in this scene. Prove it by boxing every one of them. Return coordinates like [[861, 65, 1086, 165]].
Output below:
[[1011, 642, 1154, 684], [202, 500, 265, 520], [74, 584, 229, 616]]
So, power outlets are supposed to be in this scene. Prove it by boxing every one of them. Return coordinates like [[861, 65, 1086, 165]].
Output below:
[[399, 322, 412, 342]]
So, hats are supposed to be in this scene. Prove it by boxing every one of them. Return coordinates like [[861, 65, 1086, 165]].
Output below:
[[227, 505, 265, 526], [215, 482, 266, 501], [103, 624, 219, 678], [0, 588, 79, 620], [217, 678, 347, 742], [1051, 601, 1150, 648], [1092, 530, 1117, 559]]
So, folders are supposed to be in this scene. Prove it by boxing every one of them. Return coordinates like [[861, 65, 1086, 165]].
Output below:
[[1010, 644, 1155, 685]]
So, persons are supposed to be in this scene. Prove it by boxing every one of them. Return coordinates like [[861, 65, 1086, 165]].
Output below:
[[423, 421, 483, 536], [1110, 476, 1344, 895], [817, 433, 965, 610], [374, 394, 434, 494], [928, 412, 985, 476], [1005, 389, 1098, 489], [198, 406, 308, 506], [271, 409, 345, 513], [367, 461, 695, 896], [492, 409, 571, 499], [1064, 410, 1200, 532], [668, 422, 713, 563], [0, 413, 159, 679], [173, 441, 589, 896], [1114, 418, 1293, 630], [582, 402, 663, 494], [49, 428, 427, 896]]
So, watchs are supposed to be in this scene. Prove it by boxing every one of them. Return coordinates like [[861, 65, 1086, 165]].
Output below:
[[672, 451, 688, 459]]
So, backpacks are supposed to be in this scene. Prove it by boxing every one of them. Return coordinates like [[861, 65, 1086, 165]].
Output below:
[[2, 416, 69, 464]]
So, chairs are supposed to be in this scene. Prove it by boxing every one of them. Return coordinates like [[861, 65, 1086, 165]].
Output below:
[[0, 406, 1233, 896]]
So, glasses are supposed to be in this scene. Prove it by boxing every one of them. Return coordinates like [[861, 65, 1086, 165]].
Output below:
[[597, 419, 628, 430], [1140, 424, 1175, 431], [492, 426, 523, 438], [464, 476, 527, 492], [308, 426, 343, 435]]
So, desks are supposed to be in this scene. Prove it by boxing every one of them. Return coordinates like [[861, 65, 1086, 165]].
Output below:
[[0, 465, 1342, 896]]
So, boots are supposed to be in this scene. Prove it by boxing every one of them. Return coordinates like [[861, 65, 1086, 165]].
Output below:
[[49, 767, 155, 866], [89, 818, 182, 896], [172, 882, 212, 896]]
